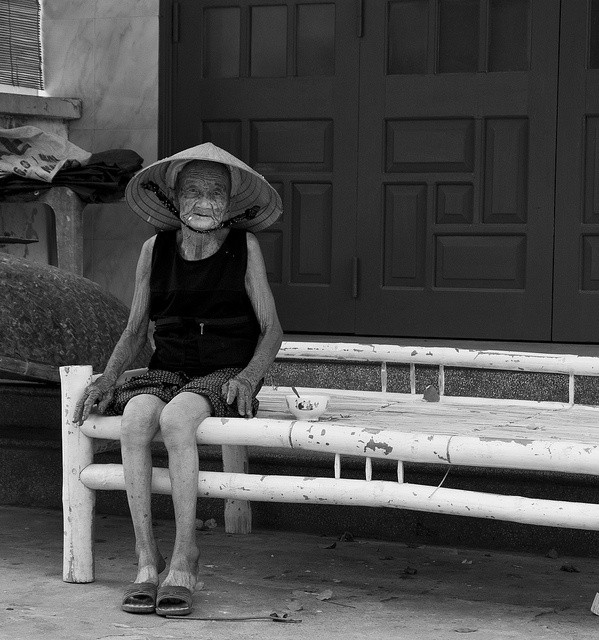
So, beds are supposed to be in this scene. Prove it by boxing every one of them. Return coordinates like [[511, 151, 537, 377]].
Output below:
[[58, 342, 599, 615]]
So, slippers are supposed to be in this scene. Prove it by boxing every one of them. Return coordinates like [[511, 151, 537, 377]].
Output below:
[[120, 584, 159, 616], [154, 584, 194, 616]]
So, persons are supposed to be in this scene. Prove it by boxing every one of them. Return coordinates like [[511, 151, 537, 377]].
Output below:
[[71, 143, 284, 616]]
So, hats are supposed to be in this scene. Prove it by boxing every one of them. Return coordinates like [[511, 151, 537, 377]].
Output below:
[[125, 143, 283, 232]]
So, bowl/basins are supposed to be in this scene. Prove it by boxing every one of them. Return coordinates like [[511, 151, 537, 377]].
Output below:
[[286, 395, 329, 422]]
